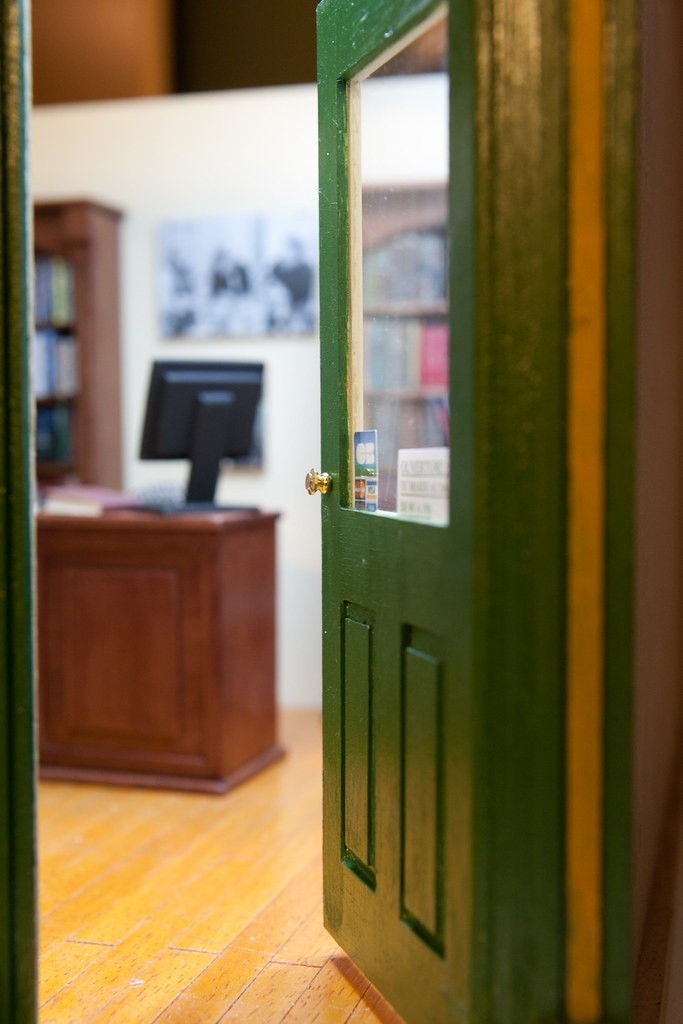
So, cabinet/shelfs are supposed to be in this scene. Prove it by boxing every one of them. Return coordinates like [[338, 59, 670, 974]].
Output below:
[[361, 185, 445, 506], [33, 199, 124, 490]]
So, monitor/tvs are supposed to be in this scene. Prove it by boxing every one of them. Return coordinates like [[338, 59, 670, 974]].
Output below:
[[127, 362, 266, 511]]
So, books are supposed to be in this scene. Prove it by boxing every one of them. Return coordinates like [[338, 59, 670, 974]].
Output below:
[[362, 321, 446, 387], [367, 401, 448, 505], [31, 335, 79, 399], [32, 259, 73, 323]]
